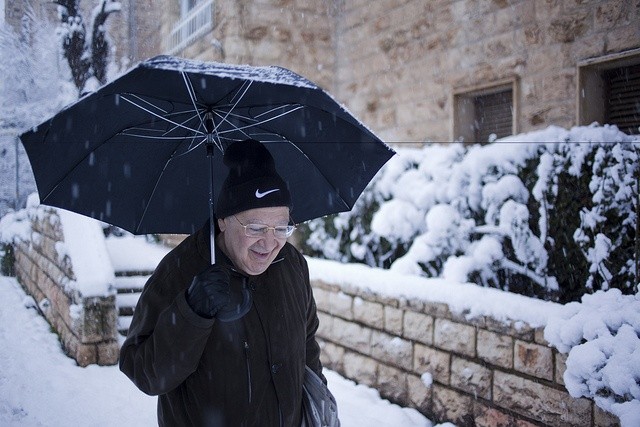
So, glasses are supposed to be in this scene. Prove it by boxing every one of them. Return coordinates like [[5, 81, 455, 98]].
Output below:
[[234, 215, 298, 241]]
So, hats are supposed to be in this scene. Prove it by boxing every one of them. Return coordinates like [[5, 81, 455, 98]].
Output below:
[[215, 136, 293, 218]]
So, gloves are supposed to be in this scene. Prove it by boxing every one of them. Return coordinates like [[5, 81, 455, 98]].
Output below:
[[184, 264, 231, 319]]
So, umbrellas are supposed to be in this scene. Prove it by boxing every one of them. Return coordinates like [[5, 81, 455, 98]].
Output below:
[[17, 56, 397, 322]]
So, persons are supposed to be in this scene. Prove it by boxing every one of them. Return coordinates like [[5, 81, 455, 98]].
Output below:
[[120, 139, 327, 427]]
